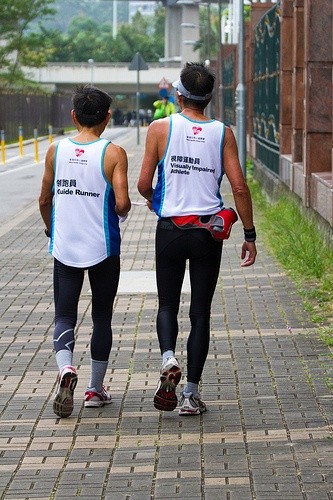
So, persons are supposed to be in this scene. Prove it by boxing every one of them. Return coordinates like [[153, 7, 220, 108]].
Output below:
[[138, 62, 258, 415], [112, 108, 124, 125], [139, 108, 152, 127], [39, 82, 131, 419], [153, 96, 174, 117], [127, 111, 132, 123]]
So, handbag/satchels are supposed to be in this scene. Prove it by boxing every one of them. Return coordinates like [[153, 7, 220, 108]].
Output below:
[[171, 206, 238, 240]]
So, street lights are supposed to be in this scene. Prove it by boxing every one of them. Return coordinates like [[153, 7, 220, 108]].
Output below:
[[88, 59, 94, 86]]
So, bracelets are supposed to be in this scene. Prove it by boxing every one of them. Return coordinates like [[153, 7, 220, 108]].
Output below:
[[45, 229, 51, 238], [243, 225, 256, 241]]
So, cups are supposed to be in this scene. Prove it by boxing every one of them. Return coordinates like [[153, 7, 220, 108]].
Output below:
[[213, 207, 239, 232]]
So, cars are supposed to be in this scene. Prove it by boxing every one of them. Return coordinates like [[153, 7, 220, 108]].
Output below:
[[111, 107, 154, 127]]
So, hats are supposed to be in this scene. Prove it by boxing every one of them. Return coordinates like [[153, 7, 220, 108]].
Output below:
[[172, 78, 213, 100]]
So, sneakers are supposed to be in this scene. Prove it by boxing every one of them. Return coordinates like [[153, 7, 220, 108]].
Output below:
[[154, 359, 182, 412], [179, 392, 206, 415], [53, 366, 77, 419], [84, 386, 113, 407]]
